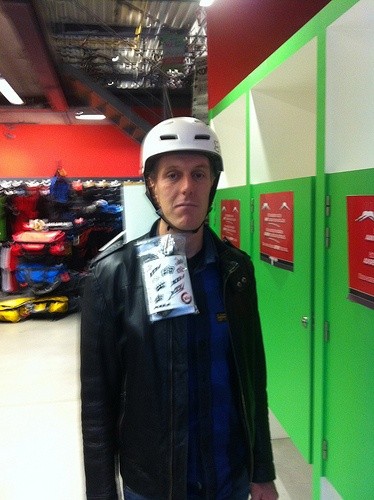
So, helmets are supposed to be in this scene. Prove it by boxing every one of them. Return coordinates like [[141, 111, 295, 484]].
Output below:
[[141, 116, 223, 185]]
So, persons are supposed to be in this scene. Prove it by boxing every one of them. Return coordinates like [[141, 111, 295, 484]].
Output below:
[[81, 117, 278, 500]]
